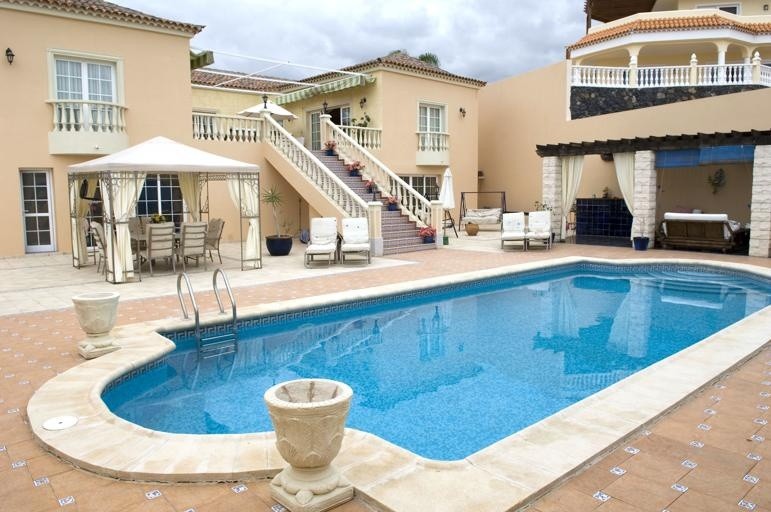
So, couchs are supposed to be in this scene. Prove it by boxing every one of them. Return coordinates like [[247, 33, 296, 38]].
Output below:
[[660, 212, 741, 253]]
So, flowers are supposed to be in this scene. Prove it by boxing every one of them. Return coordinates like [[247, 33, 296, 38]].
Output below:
[[321, 140, 338, 151], [419, 223, 436, 239], [384, 195, 401, 206]]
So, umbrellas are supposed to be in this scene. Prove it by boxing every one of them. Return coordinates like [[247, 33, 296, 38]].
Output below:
[[238, 98, 299, 121], [438, 167, 456, 236]]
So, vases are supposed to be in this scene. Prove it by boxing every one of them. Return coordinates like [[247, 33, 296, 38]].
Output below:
[[633, 236, 650, 250], [71, 291, 121, 359], [424, 236, 435, 243], [325, 150, 334, 156], [260, 378, 365, 512], [389, 204, 398, 211]]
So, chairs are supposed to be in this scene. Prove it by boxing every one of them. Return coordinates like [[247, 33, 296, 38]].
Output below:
[[89, 214, 227, 277], [501, 210, 553, 251], [303, 216, 373, 268]]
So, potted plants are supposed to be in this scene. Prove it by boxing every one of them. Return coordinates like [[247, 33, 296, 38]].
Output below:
[[260, 182, 294, 256]]
